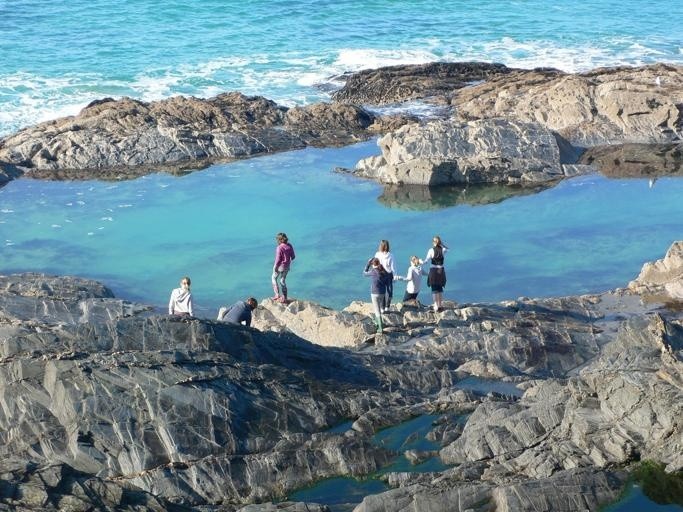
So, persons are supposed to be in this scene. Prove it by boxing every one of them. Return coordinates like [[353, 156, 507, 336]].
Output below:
[[168, 277, 194, 318], [363, 257, 390, 334], [418, 236, 449, 312], [374, 239, 398, 314], [398, 255, 430, 308], [216, 297, 257, 327], [269, 233, 296, 303]]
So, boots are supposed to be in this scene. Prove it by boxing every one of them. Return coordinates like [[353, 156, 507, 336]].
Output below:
[[271, 286, 280, 300], [376, 316, 383, 333], [280, 288, 287, 302]]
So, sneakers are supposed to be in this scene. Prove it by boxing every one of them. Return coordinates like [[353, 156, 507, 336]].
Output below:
[[434, 307, 441, 312], [381, 309, 390, 314]]
